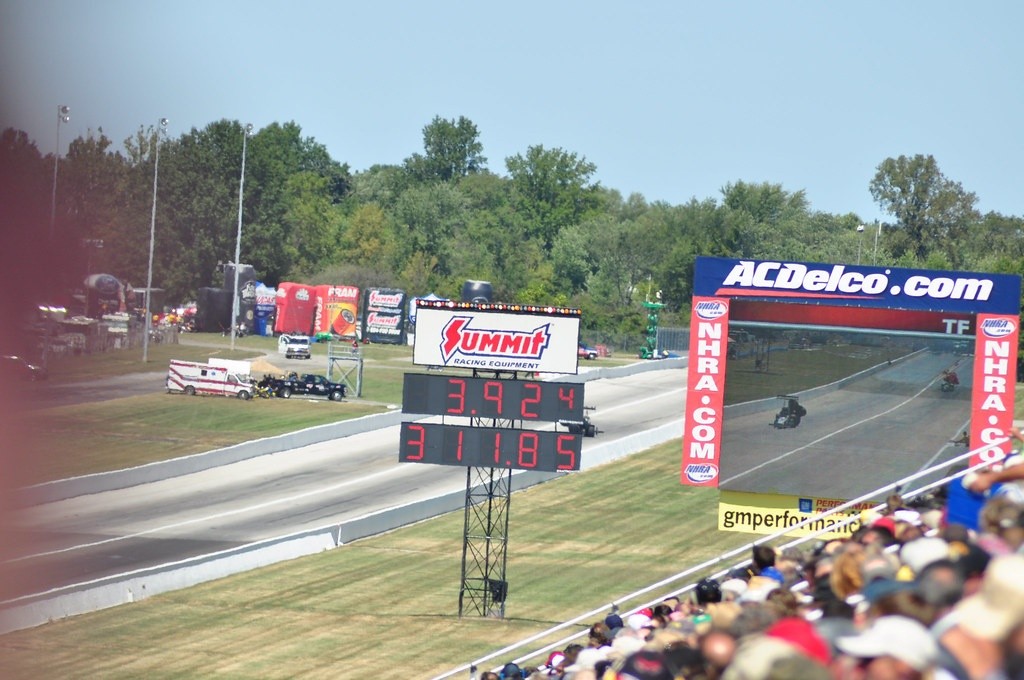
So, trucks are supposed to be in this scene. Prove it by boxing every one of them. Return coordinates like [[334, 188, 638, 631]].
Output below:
[[167, 360, 253, 400]]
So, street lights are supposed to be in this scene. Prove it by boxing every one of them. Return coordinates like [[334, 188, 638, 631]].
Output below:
[[48, 104, 69, 233], [142, 118, 171, 363], [230, 122, 254, 349], [856, 225, 864, 265]]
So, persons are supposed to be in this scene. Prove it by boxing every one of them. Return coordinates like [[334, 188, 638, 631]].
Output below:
[[950, 432, 969, 448], [246, 373, 279, 400], [352, 340, 358, 355], [284, 370, 298, 381], [481, 425, 1024, 680]]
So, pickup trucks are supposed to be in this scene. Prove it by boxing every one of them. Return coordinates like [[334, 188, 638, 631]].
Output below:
[[267, 374, 348, 401]]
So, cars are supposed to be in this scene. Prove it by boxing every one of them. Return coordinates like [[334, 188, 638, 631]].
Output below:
[[101, 314, 130, 337], [146, 330, 164, 343], [577, 342, 599, 361]]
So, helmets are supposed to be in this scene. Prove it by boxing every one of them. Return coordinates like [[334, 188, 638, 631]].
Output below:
[[546, 652, 566, 676], [697, 578, 722, 606]]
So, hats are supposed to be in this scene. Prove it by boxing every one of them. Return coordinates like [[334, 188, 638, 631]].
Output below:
[[564, 648, 609, 672], [835, 615, 940, 673], [717, 578, 748, 597], [899, 536, 949, 575], [606, 636, 643, 658], [893, 511, 922, 526], [1002, 449, 1023, 469], [619, 652, 675, 680], [761, 566, 784, 584], [606, 612, 623, 629], [765, 614, 832, 667], [951, 552, 1024, 641], [918, 509, 943, 532], [624, 614, 652, 630], [501, 663, 520, 678], [873, 516, 895, 539]]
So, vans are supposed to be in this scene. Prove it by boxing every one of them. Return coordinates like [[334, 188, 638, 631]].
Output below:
[[278, 334, 311, 359]]
